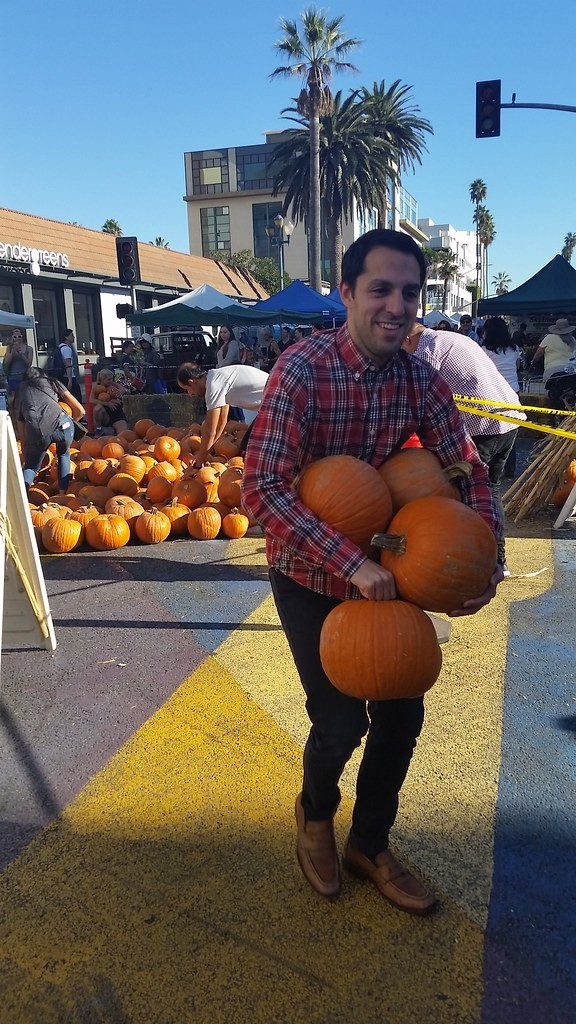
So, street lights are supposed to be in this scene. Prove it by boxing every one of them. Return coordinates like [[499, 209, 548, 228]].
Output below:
[[264, 213, 294, 291]]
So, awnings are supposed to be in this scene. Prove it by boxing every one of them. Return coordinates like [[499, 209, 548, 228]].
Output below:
[[0, 310, 36, 330]]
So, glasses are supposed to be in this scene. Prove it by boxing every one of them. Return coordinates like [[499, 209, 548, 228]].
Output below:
[[439, 327, 448, 330], [461, 321, 470, 324], [12, 335, 23, 339]]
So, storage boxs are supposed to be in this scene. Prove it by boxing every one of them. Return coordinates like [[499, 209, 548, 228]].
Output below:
[[520, 393, 550, 425], [523, 382, 549, 394]]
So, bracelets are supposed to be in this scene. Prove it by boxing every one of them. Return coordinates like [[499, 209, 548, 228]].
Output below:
[[193, 462, 202, 469]]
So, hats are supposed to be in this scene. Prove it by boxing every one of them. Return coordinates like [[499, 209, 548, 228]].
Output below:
[[460, 315, 471, 321], [137, 334, 152, 346], [548, 319, 576, 334]]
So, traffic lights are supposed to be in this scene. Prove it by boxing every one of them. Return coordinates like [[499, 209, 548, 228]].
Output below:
[[116, 236, 142, 285], [475, 80, 501, 138], [116, 302, 133, 319]]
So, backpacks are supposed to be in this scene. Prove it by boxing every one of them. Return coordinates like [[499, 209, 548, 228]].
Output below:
[[45, 345, 67, 379]]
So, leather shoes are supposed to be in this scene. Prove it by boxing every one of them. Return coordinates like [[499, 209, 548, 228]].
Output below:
[[342, 833, 436, 914], [295, 790, 341, 897]]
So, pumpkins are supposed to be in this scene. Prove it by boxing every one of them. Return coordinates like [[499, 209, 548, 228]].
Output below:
[[292, 454, 392, 554], [14, 385, 259, 553], [546, 459, 576, 507], [319, 598, 442, 701], [370, 496, 497, 613], [375, 446, 473, 515]]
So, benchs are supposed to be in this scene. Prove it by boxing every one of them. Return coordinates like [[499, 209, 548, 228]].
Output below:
[[110, 337, 138, 361], [163, 341, 200, 365]]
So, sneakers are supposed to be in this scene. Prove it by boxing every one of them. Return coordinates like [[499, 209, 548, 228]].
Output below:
[[95, 427, 105, 438]]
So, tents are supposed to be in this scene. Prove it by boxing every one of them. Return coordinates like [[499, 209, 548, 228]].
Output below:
[[126, 277, 464, 325], [476, 253, 576, 318]]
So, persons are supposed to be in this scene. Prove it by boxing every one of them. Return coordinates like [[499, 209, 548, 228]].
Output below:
[[241, 226, 506, 915], [433, 314, 576, 429], [177, 361, 269, 480], [400, 320, 528, 576], [214, 324, 325, 376], [43, 328, 83, 407], [135, 333, 169, 394], [89, 369, 128, 438], [3, 329, 34, 404], [13, 367, 87, 495], [119, 341, 144, 395]]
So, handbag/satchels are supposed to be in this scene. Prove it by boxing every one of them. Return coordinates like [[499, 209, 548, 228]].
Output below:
[[3, 345, 11, 373], [132, 376, 146, 391], [229, 360, 242, 365], [114, 368, 137, 391], [70, 418, 88, 442], [154, 378, 167, 394]]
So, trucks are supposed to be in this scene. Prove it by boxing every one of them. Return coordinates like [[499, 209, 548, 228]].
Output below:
[[101, 330, 217, 395]]
